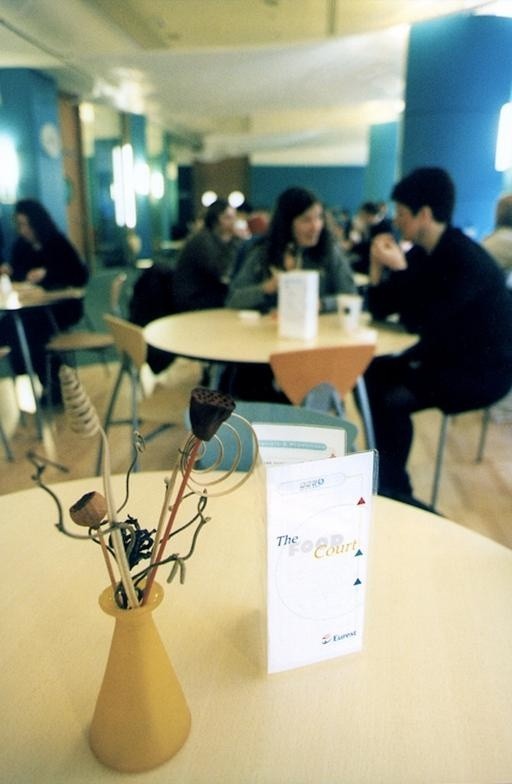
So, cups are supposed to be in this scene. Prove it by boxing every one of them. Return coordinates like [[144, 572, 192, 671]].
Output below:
[[336, 295, 362, 330]]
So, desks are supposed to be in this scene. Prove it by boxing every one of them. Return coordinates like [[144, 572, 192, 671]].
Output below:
[[0, 471, 511, 783], [144, 308, 420, 451], [0, 282, 86, 442]]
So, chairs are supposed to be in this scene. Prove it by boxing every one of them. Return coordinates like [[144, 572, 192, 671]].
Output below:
[[185, 402, 358, 472], [273, 346, 377, 420], [45, 272, 127, 426], [94, 314, 186, 477]]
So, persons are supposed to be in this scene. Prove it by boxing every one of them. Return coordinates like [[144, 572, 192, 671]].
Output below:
[[1, 198, 91, 412], [482, 193, 512, 290], [211, 187, 357, 404], [323, 200, 402, 298], [352, 166, 511, 518], [175, 200, 254, 311]]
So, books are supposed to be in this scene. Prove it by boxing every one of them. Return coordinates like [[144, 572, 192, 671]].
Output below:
[[262, 450, 378, 679]]
